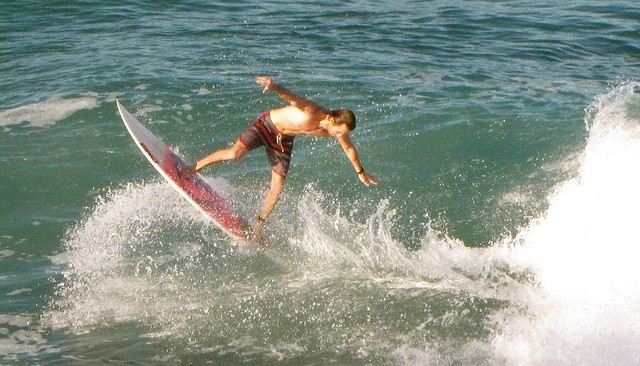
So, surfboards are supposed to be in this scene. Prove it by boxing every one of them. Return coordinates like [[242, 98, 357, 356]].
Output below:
[[116, 98, 254, 240]]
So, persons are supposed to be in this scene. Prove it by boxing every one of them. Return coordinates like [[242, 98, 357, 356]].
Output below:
[[178, 75, 379, 240]]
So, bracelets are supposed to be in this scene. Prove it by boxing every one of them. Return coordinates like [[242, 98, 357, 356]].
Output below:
[[358, 167, 364, 174]]
